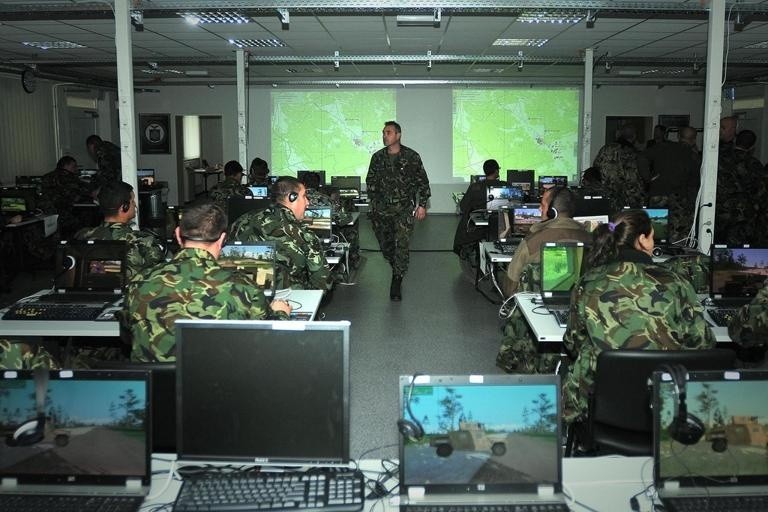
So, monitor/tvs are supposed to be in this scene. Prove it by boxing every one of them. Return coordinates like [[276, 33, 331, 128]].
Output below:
[[538, 176, 568, 197], [268, 176, 279, 184], [297, 171, 325, 185], [138, 169, 154, 176], [300, 206, 332, 244], [243, 184, 268, 196], [485, 186, 522, 213], [511, 205, 541, 236], [476, 175, 487, 180], [227, 199, 271, 225], [0, 195, 29, 214], [331, 175, 360, 199], [78, 169, 97, 183], [174, 319, 350, 465], [573, 215, 609, 232], [709, 244, 768, 300], [622, 206, 670, 246], [507, 169, 534, 193]]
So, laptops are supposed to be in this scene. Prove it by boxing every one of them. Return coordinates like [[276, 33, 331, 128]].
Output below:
[[0, 369, 151, 512], [541, 241, 585, 311], [398, 374, 570, 512], [36, 240, 127, 305], [217, 241, 276, 304], [651, 370, 768, 512]]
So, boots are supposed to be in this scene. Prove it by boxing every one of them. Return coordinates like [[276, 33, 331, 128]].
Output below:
[[390, 276, 402, 302]]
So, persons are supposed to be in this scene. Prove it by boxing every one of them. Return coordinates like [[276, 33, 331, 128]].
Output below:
[[75, 180, 166, 280], [37, 156, 97, 213], [720, 117, 737, 148], [209, 161, 253, 200], [563, 208, 716, 436], [87, 134, 121, 178], [595, 123, 646, 204], [728, 278, 768, 345], [302, 172, 352, 225], [678, 126, 700, 160], [647, 125, 673, 147], [716, 130, 764, 245], [366, 121, 431, 300], [121, 202, 292, 363], [461, 159, 511, 214], [228, 176, 338, 296], [502, 186, 590, 327], [245, 158, 270, 186]]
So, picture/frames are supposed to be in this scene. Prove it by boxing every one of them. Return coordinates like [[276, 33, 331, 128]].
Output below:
[[139, 112, 171, 155]]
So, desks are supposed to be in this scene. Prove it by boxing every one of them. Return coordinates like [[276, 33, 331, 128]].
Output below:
[[195, 168, 223, 192], [72, 200, 99, 207], [483, 240, 672, 263], [320, 242, 351, 264], [141, 450, 664, 511], [331, 211, 361, 226], [453, 191, 466, 203], [353, 198, 371, 206], [470, 211, 490, 226], [515, 290, 733, 342], [0, 290, 325, 337]]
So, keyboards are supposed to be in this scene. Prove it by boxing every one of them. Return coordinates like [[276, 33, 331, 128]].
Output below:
[[707, 305, 736, 326], [554, 309, 571, 328], [500, 239, 520, 254], [171, 466, 365, 511], [1, 302, 100, 323]]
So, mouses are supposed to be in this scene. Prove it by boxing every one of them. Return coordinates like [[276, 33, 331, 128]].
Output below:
[[653, 247, 663, 258], [327, 250, 334, 257]]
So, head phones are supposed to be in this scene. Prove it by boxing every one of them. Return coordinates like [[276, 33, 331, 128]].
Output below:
[[656, 362, 704, 446], [582, 179, 584, 184], [488, 187, 495, 201], [59, 241, 75, 270], [4, 370, 49, 448], [104, 201, 130, 212], [545, 188, 561, 220], [290, 192, 298, 202], [396, 373, 425, 442]]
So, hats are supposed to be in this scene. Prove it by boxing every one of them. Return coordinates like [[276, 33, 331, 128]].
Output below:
[[483, 160, 501, 175]]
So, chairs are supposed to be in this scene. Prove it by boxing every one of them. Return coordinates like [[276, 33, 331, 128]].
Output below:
[[89, 359, 177, 449], [584, 347, 736, 455], [73, 240, 130, 290]]
